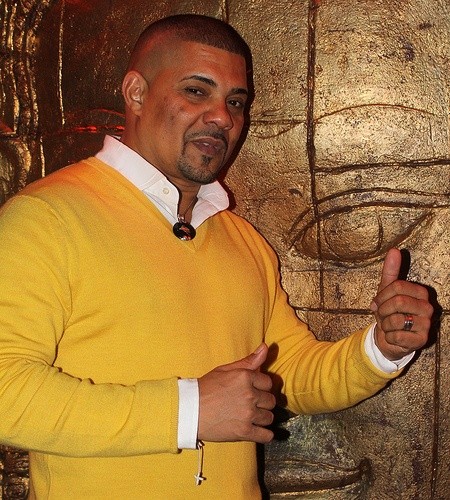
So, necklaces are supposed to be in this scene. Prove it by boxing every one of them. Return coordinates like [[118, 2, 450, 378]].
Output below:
[[172, 193, 203, 241]]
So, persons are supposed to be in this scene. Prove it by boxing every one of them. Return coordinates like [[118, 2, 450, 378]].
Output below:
[[3, 13, 441, 500]]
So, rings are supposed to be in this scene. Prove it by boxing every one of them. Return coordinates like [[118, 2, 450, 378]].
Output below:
[[402, 314, 415, 332]]
